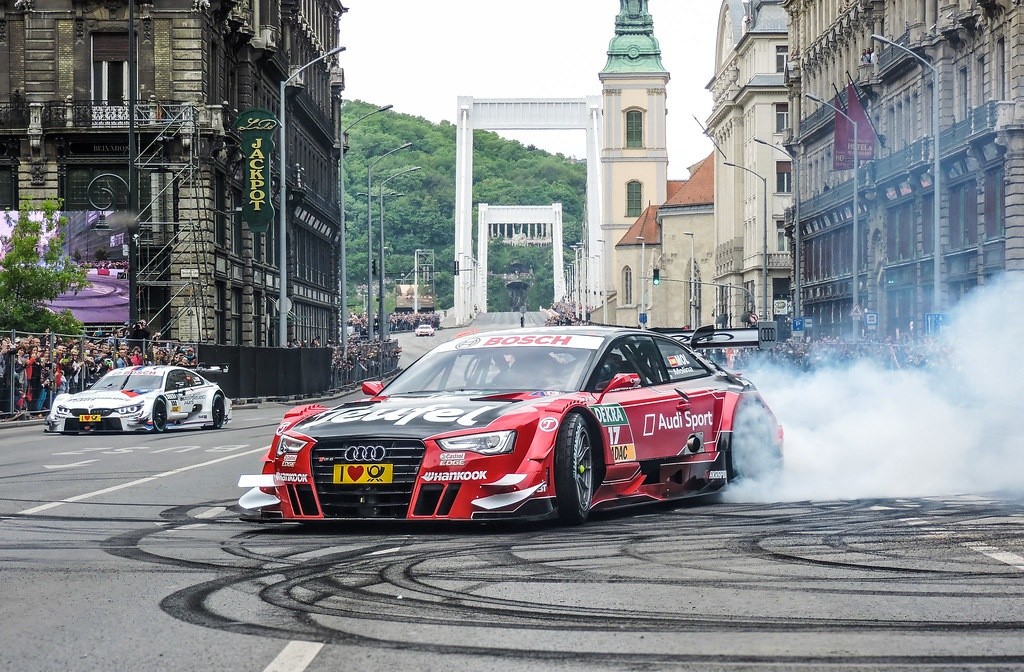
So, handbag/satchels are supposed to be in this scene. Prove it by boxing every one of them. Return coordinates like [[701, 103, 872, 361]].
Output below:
[[14, 362, 23, 372]]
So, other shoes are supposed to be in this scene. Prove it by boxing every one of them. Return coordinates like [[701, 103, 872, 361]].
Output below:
[[38, 414, 42, 418]]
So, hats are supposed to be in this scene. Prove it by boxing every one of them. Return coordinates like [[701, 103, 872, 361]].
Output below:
[[26, 345, 38, 354], [187, 348, 193, 352]]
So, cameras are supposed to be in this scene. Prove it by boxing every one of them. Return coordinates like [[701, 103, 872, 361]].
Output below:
[[135, 321, 142, 329]]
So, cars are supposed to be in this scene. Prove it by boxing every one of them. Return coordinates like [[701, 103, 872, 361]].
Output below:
[[415, 324, 435, 336]]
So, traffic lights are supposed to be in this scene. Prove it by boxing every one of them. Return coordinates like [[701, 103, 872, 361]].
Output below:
[[653, 269, 659, 285]]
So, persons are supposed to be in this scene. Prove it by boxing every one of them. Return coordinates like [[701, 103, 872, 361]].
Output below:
[[545, 298, 591, 327], [287, 309, 441, 399], [0, 319, 197, 418], [698, 334, 945, 374]]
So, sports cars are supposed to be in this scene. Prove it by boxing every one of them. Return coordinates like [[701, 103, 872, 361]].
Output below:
[[43, 365, 232, 435], [239, 325, 784, 528]]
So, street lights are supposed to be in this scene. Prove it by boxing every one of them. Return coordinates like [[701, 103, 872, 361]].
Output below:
[[570, 242, 588, 323], [684, 231, 696, 330], [806, 93, 860, 341], [457, 251, 481, 326], [724, 161, 769, 322], [596, 239, 608, 325], [870, 34, 942, 311], [379, 165, 423, 341], [368, 144, 411, 343], [279, 46, 348, 346], [754, 138, 803, 344], [341, 105, 393, 345], [635, 237, 646, 330]]
